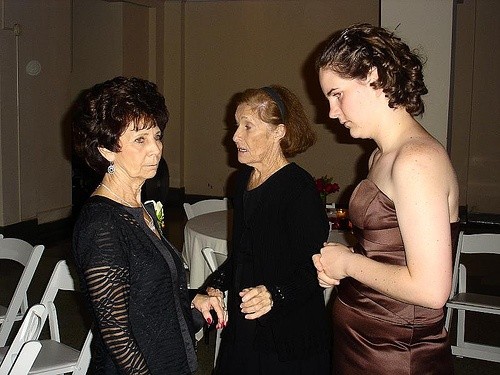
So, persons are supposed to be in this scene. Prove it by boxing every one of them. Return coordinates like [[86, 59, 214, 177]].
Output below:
[[199, 85, 329, 375], [72, 77, 228, 375], [312, 23, 461, 375]]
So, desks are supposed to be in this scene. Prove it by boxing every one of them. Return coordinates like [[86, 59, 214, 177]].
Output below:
[[181, 208, 360, 307]]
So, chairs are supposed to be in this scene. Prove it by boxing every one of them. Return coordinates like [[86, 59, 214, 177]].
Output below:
[[0, 233, 45, 348], [444, 230, 500, 364], [0, 260, 107, 375]]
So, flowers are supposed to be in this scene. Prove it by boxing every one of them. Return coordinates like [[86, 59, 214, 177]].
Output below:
[[313, 175, 340, 195]]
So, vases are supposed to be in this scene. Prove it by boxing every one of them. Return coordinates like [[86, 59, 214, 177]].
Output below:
[[320, 195, 326, 209]]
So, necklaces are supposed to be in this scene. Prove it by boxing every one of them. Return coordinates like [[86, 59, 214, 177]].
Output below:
[[100, 182, 156, 231]]
